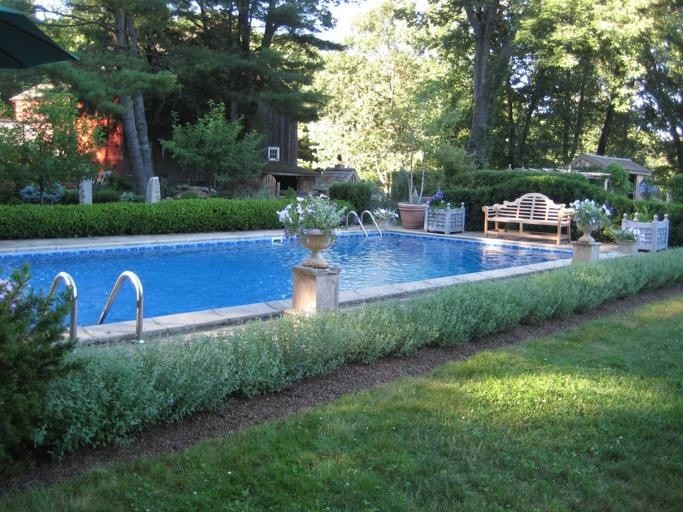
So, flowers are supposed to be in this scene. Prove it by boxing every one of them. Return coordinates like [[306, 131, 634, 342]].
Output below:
[[569, 198, 614, 232], [373, 206, 399, 221], [275, 193, 349, 250], [611, 227, 640, 241], [427, 191, 460, 216]]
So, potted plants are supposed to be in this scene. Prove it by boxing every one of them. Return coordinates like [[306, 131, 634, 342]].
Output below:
[[621, 212, 670, 253]]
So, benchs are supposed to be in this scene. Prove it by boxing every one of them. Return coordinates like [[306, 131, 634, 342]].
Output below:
[[482, 193, 575, 247]]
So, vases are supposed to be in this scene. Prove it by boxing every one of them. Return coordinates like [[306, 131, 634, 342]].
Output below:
[[398, 201, 426, 229], [300, 227, 337, 269], [423, 201, 465, 234], [575, 222, 599, 243], [616, 239, 639, 253]]
